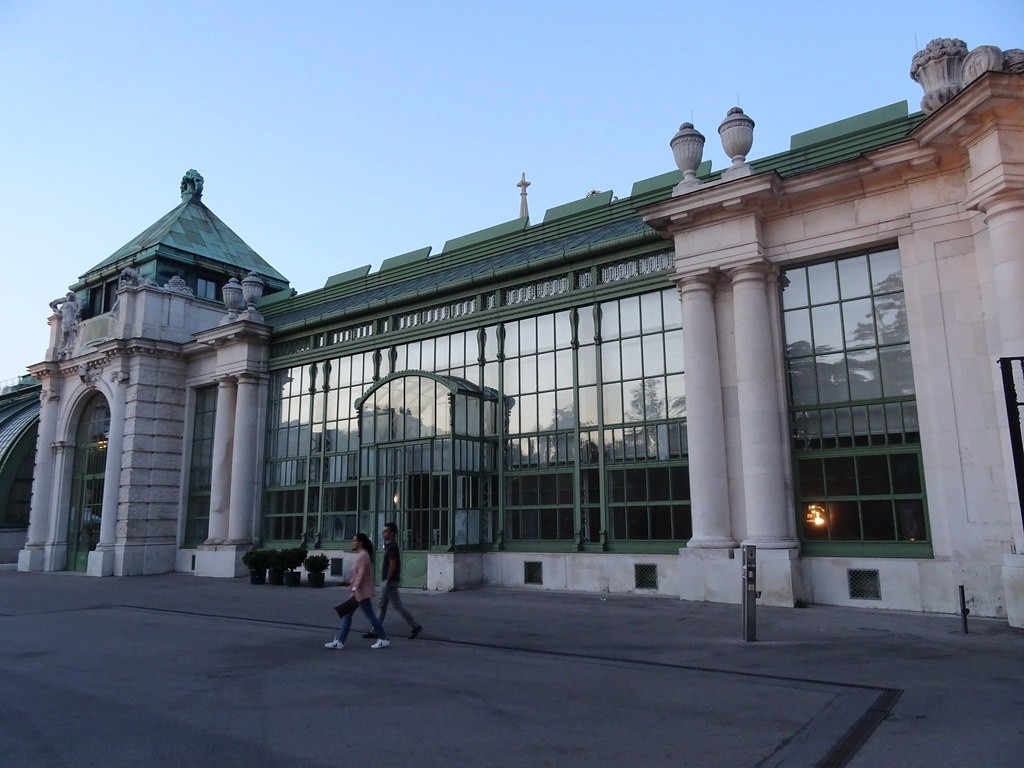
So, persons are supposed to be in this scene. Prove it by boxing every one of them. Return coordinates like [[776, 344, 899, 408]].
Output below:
[[325, 533, 392, 649], [361, 522, 422, 639]]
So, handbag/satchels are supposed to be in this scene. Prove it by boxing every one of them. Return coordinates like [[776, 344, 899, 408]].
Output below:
[[334, 596, 360, 619]]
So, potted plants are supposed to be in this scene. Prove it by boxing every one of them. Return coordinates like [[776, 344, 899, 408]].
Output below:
[[303, 553, 331, 589], [281, 547, 308, 586], [242, 550, 268, 584], [266, 549, 285, 587]]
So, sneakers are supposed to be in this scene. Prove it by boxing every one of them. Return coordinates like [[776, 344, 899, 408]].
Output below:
[[371, 638, 390, 648], [324, 640, 345, 649]]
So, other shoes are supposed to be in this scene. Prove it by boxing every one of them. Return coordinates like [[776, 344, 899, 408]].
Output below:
[[361, 631, 379, 639], [408, 625, 422, 639]]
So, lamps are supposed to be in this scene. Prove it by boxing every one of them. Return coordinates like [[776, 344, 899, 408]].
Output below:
[[77, 367, 96, 389], [805, 503, 828, 526]]
